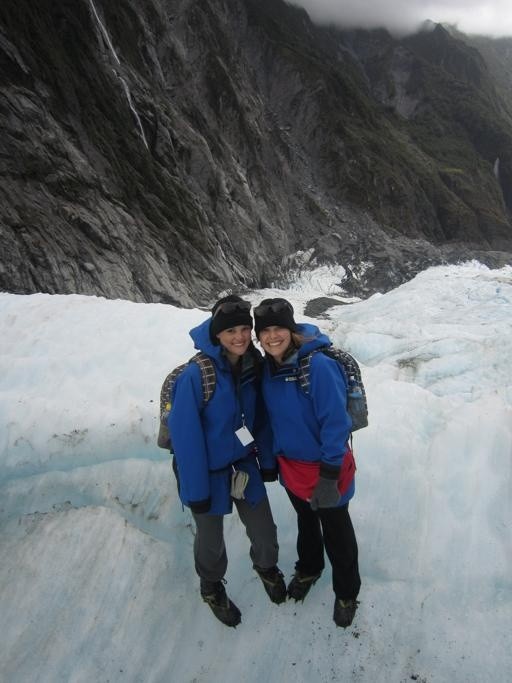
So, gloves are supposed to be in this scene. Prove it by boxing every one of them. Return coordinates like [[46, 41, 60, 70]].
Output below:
[[306, 477, 341, 511]]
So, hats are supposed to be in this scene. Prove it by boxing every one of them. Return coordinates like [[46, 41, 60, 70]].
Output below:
[[253, 298, 296, 341], [211, 295, 253, 336]]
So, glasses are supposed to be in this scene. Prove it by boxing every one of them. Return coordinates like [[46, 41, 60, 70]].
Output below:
[[253, 301, 292, 317], [211, 301, 252, 322]]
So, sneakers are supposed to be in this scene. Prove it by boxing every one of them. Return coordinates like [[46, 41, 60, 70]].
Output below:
[[333, 596, 360, 627], [257, 565, 287, 604], [201, 586, 242, 629], [287, 570, 322, 605]]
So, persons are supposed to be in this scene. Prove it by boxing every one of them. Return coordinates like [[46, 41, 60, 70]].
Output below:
[[252, 298, 362, 628], [167, 295, 286, 628]]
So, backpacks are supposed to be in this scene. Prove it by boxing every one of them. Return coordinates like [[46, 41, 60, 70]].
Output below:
[[157, 353, 216, 450], [297, 347, 368, 432]]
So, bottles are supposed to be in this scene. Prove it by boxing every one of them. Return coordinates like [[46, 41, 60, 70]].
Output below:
[[157, 402, 174, 449]]
